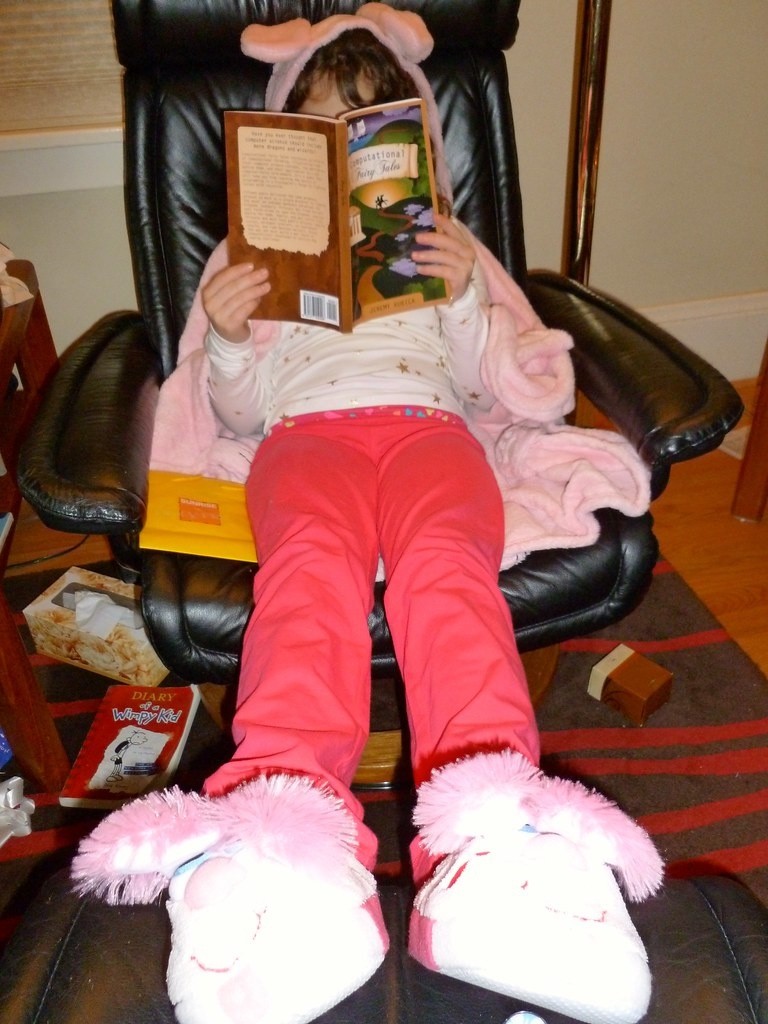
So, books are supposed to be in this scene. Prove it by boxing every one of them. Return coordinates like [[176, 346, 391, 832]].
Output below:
[[225, 98, 453, 332], [57, 684, 201, 810]]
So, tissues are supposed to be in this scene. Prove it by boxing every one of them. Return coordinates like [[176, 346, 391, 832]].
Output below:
[[22, 566, 173, 690]]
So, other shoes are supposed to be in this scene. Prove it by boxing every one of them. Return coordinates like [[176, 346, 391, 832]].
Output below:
[[406, 746, 666, 1024], [69, 773, 389, 1024]]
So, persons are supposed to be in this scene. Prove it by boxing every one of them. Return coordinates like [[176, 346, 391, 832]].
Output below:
[[72, 1, 667, 1024]]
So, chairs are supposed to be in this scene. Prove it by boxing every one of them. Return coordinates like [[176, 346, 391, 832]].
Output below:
[[18, 0, 745, 684]]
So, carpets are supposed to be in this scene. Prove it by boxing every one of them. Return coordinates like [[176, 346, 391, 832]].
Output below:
[[0, 559, 768, 940]]
